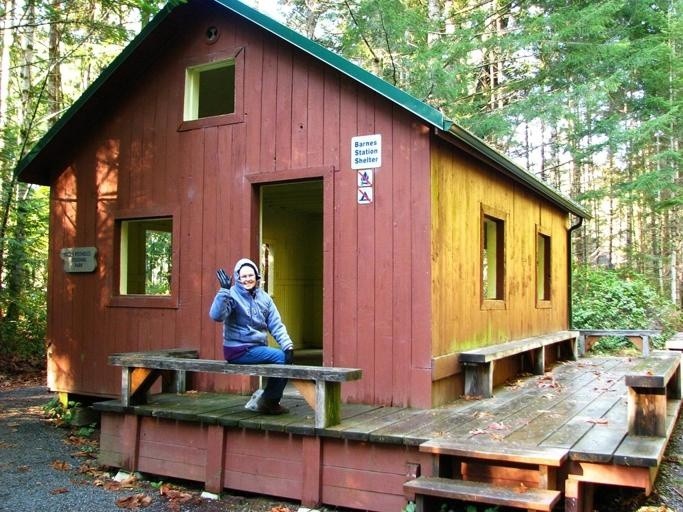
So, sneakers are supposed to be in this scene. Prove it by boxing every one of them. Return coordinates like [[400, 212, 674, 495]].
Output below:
[[257, 399, 288, 414]]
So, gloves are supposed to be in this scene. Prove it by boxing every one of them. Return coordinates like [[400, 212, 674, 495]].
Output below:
[[284, 349, 293, 363], [215, 268, 231, 288]]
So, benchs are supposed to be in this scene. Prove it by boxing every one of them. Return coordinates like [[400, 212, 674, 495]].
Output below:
[[460, 327, 682, 438], [108, 348, 361, 431]]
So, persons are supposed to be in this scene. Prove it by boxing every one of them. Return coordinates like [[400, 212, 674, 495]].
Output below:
[[209, 258, 294, 415]]
